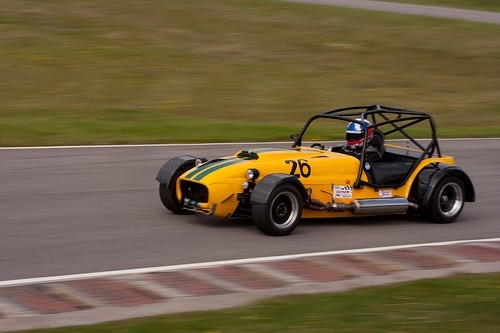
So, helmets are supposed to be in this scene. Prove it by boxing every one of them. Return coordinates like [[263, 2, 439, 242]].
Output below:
[[346, 119, 373, 149]]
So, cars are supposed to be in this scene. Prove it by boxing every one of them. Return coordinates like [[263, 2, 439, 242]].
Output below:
[[155, 105, 477, 236]]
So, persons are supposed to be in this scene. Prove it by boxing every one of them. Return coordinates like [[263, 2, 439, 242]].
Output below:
[[328, 119, 382, 164]]
